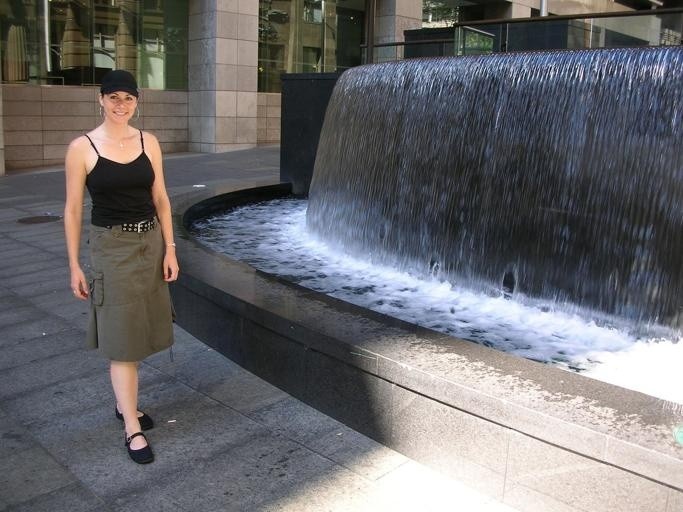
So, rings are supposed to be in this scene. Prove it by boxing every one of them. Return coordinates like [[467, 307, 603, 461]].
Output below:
[[73, 290, 76, 294]]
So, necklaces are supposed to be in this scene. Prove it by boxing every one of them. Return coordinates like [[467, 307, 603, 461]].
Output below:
[[99, 123, 131, 152]]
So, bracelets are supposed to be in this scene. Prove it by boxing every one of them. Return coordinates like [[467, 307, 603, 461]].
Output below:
[[166, 241, 176, 248]]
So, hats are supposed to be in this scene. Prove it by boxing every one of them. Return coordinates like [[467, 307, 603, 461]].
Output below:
[[99, 70, 140, 98]]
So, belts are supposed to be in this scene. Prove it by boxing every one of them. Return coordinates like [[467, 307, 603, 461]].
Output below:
[[104, 215, 160, 235]]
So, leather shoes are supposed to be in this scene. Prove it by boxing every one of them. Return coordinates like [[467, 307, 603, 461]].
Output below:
[[124, 431, 155, 465], [114, 404, 154, 431]]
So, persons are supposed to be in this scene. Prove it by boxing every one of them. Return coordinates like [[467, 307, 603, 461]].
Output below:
[[64, 70, 180, 465]]
